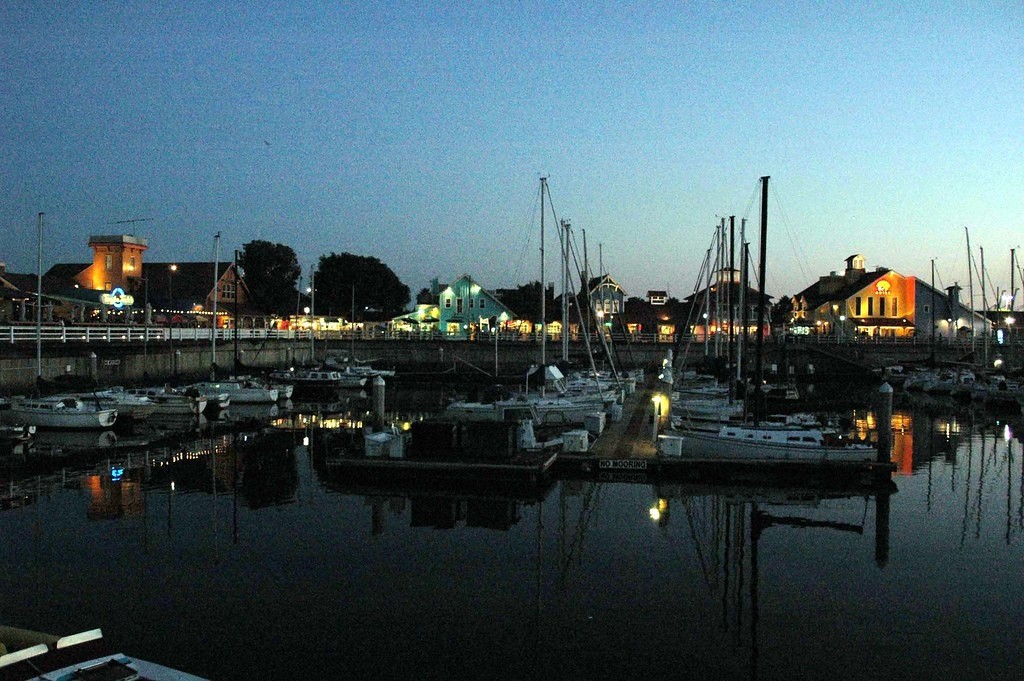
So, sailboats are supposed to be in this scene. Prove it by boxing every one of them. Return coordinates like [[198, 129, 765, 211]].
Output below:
[[0, 174, 1023, 478]]
